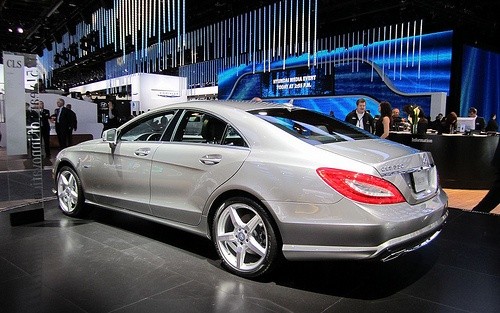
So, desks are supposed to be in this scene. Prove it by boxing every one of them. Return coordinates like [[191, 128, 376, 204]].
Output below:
[[390, 130, 500, 174], [24, 133, 93, 170]]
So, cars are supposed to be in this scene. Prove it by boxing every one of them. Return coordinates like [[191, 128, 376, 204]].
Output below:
[[51, 99, 449, 280]]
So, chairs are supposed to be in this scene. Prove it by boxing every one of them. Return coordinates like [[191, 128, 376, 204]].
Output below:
[[174, 115, 203, 143]]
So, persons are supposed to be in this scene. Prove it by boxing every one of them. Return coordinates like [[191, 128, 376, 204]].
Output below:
[[403, 105, 427, 133], [428, 110, 460, 134], [345, 98, 375, 134], [84, 91, 93, 103], [26, 102, 31, 150], [375, 101, 392, 140], [50, 98, 73, 150], [147, 109, 168, 128], [392, 107, 406, 131], [34, 79, 46, 92], [31, 100, 51, 156], [469, 108, 485, 130], [66, 103, 77, 147], [47, 79, 50, 87], [104, 109, 143, 130], [330, 111, 335, 118], [485, 112, 499, 132]]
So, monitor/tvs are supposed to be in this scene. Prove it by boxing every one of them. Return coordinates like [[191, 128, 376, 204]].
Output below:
[[457, 117, 475, 132]]
